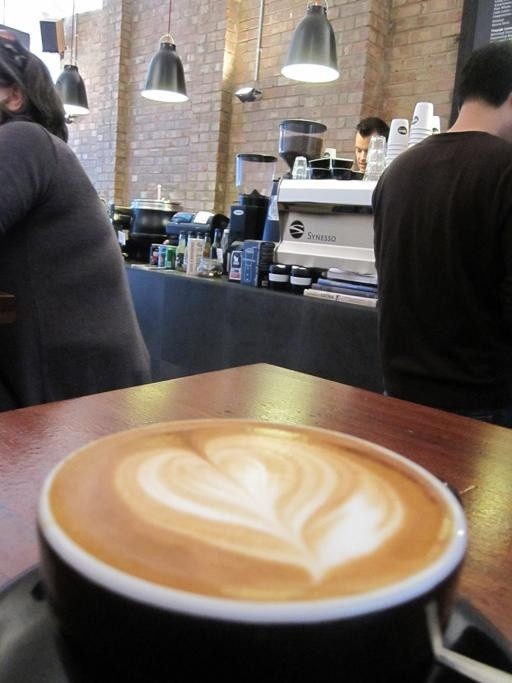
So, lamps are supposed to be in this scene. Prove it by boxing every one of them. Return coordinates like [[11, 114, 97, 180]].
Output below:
[[54, 0, 90, 115], [235, 0, 265, 104], [140, 0, 189, 104], [281, 1, 341, 84]]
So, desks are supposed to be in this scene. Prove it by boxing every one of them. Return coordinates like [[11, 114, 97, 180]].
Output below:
[[0, 361, 512, 683]]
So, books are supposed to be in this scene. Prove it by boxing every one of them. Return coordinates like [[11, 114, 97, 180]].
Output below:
[[304, 267, 378, 308]]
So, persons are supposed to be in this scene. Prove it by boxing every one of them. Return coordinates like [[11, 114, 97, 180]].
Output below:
[[372, 39, 511, 430], [356, 117, 389, 172], [0, 36, 151, 414]]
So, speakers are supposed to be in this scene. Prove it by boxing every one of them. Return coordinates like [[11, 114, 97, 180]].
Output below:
[[40, 18, 65, 54]]
